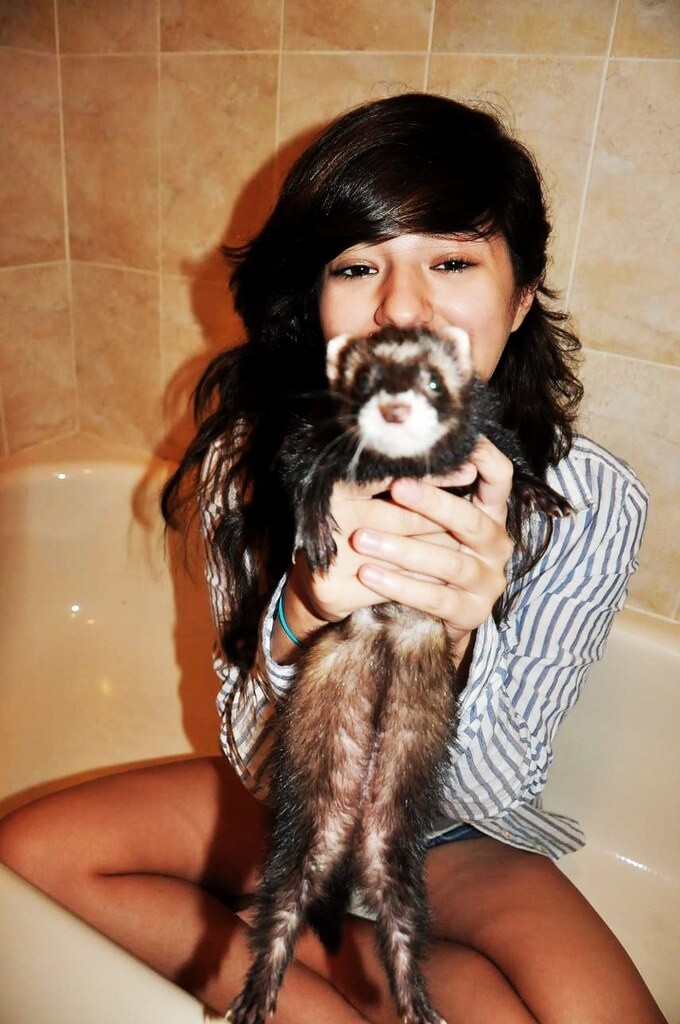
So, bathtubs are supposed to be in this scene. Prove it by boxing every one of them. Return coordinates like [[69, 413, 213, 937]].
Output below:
[[0, 427, 679, 1024]]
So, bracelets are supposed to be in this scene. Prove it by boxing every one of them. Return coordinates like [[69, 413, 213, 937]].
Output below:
[[278, 598, 304, 649]]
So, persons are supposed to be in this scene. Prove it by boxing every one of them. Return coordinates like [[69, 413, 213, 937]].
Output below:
[[0, 94, 668, 1023]]
